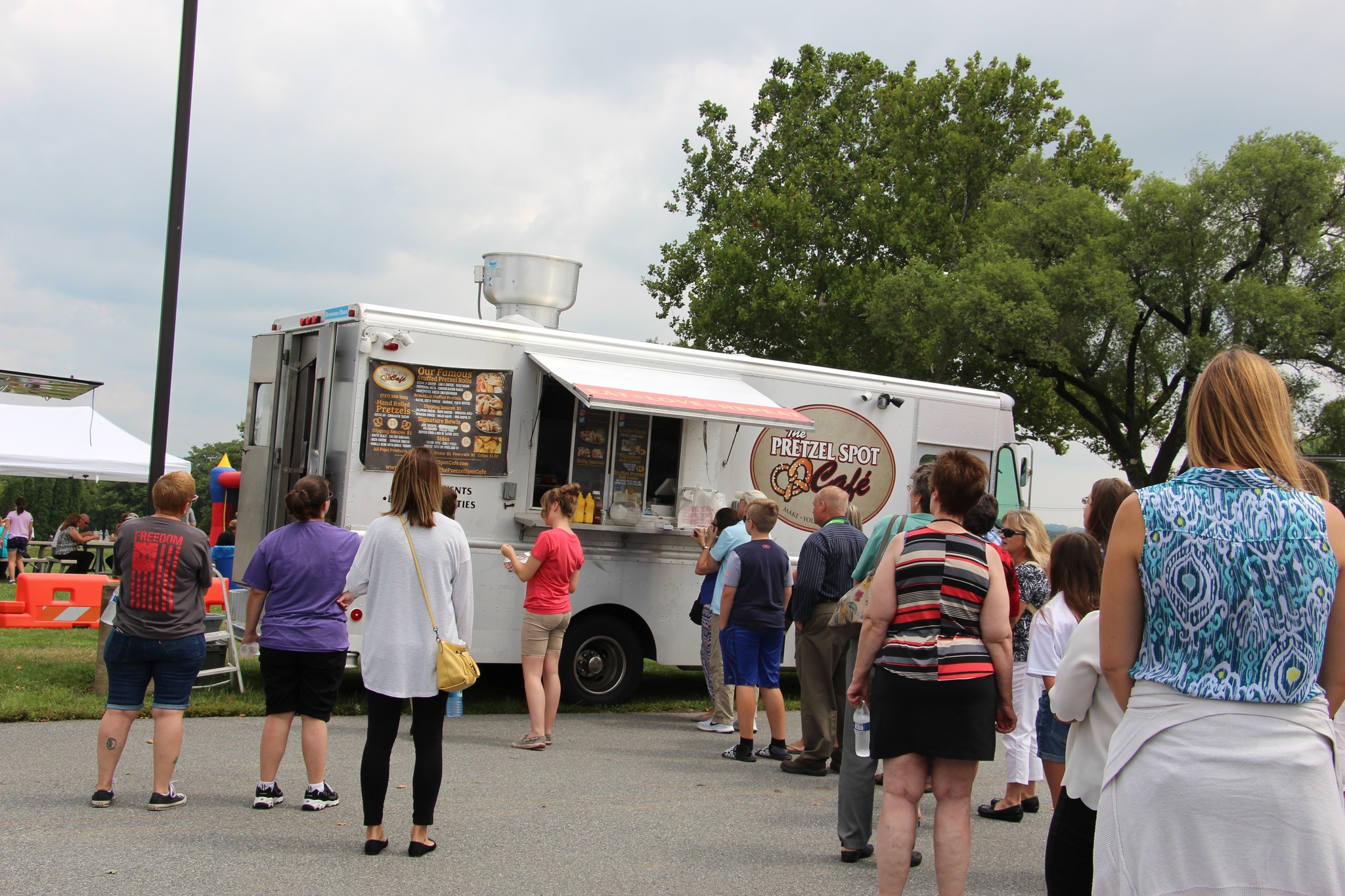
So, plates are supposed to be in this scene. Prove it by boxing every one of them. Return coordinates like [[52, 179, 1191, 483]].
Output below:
[[580, 431, 605, 444], [476, 420, 502, 434]]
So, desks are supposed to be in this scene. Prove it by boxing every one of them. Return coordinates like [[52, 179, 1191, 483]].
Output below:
[[81, 541, 115, 574], [28, 541, 53, 573]]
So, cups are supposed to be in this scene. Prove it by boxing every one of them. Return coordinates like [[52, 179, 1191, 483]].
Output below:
[[636, 445, 640, 452], [93, 531, 99, 535], [600, 511, 606, 525]]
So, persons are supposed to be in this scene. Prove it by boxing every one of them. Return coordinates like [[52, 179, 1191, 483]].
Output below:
[[105, 512, 139, 578], [216, 519, 237, 577], [91, 470, 212, 811], [500, 483, 584, 749], [240, 474, 363, 811], [696, 490, 772, 733], [690, 507, 739, 721], [838, 447, 1050, 896], [779, 485, 868, 777], [49, 535, 54, 546], [51, 513, 95, 574], [1045, 609, 1123, 896], [0, 497, 33, 584], [0, 514, 9, 579], [410, 486, 457, 734], [14, 526, 35, 579], [1025, 533, 1104, 809], [1090, 349, 1345, 895], [1082, 478, 1133, 556], [181, 507, 196, 527], [346, 447, 475, 857], [1297, 459, 1330, 503], [719, 497, 794, 762]]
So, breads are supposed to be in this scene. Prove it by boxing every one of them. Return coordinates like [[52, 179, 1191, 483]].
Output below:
[[373, 417, 383, 427], [401, 420, 412, 430]]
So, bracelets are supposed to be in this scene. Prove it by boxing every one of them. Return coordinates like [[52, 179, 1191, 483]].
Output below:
[[702, 546, 711, 550]]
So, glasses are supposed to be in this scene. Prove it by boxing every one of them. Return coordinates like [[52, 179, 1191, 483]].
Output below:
[[190, 494, 199, 503], [127, 514, 138, 518], [710, 520, 718, 528], [81, 518, 89, 525], [326, 491, 333, 501], [1081, 496, 1093, 507], [743, 514, 756, 526], [1000, 528, 1026, 538]]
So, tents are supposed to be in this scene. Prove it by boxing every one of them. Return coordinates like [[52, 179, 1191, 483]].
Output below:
[[0, 404, 192, 526]]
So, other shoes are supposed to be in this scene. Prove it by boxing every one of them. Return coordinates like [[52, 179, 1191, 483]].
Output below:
[[841, 844, 874, 862], [408, 838, 437, 857], [6, 580, 16, 584], [923, 775, 933, 793], [691, 714, 712, 721], [365, 837, 389, 855], [978, 795, 1040, 822], [873, 772, 884, 784], [910, 851, 922, 867]]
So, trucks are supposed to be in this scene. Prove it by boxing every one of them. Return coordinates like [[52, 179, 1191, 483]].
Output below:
[[231, 260, 1034, 710]]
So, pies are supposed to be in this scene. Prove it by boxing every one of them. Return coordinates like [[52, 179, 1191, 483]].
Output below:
[[475, 373, 504, 415], [578, 447, 604, 458], [477, 419, 500, 431]]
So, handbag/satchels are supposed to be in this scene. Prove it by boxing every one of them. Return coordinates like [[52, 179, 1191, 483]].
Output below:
[[827, 514, 907, 639], [435, 637, 481, 692], [689, 600, 703, 626]]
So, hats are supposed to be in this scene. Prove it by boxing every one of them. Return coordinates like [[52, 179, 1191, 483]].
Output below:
[[734, 490, 768, 505], [124, 513, 139, 521]]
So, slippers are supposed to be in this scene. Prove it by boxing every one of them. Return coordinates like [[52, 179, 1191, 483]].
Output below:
[[755, 743, 792, 761], [722, 743, 756, 762], [786, 743, 804, 755]]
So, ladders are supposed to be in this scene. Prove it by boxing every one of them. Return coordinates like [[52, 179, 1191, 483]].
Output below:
[[192, 566, 245, 695]]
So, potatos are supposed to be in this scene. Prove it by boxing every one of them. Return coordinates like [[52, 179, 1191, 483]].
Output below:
[[473, 436, 502, 454]]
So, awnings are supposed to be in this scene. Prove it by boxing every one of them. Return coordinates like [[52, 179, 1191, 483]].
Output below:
[[526, 350, 817, 434]]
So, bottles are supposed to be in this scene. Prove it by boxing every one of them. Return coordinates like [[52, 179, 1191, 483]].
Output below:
[[99, 530, 103, 541], [105, 530, 109, 542], [585, 493, 595, 523], [609, 493, 642, 527], [573, 492, 585, 523], [592, 491, 602, 525], [100, 586, 120, 626], [446, 690, 463, 717], [239, 641, 260, 657], [853, 700, 870, 757]]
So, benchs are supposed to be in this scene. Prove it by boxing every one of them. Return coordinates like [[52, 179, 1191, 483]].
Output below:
[[0, 558, 49, 573], [46, 556, 77, 573]]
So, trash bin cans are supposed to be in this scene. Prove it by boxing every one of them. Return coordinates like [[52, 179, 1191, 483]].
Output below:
[[213, 545, 247, 590], [201, 613, 226, 634]]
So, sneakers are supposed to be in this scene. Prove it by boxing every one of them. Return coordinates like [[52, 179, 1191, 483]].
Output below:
[[302, 780, 339, 811], [697, 719, 758, 734], [148, 779, 187, 811], [253, 777, 284, 809], [512, 731, 552, 749], [780, 758, 840, 777], [91, 778, 117, 807]]
